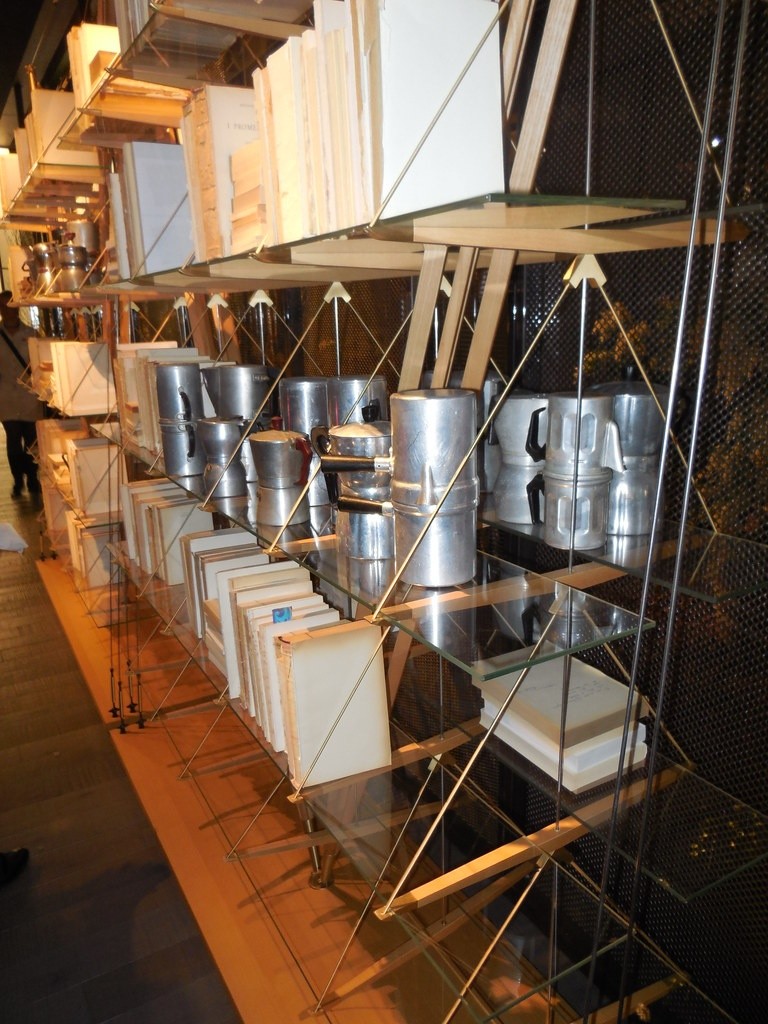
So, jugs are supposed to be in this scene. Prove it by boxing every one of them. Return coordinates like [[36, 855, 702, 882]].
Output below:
[[28, 231, 102, 272], [309, 404, 391, 497], [248, 417, 311, 488]]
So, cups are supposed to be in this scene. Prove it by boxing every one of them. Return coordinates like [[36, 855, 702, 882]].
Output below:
[[527, 389, 626, 552], [153, 359, 208, 478]]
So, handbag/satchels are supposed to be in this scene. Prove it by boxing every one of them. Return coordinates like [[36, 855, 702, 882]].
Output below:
[[45, 404, 60, 419]]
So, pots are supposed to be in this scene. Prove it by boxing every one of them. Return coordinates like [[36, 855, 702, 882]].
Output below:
[[318, 386, 479, 587], [590, 363, 690, 459]]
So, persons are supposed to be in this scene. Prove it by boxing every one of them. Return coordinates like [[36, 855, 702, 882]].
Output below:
[[0, 290, 44, 499]]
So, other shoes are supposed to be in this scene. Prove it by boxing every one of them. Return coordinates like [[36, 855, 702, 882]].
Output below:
[[29, 482, 41, 492], [13, 483, 23, 496], [0, 848, 29, 887]]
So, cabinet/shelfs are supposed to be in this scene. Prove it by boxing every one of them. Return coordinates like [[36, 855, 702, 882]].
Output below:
[[0, 0, 768, 1024]]
